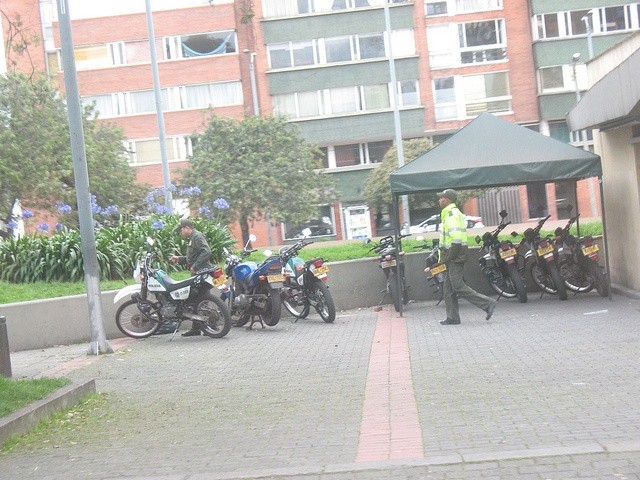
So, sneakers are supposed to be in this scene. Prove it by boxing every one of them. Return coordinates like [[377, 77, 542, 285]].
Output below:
[[486, 299, 497, 320], [440, 317, 460, 325]]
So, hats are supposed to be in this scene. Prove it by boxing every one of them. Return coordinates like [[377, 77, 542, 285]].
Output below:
[[436, 189, 457, 201], [173, 218, 193, 231]]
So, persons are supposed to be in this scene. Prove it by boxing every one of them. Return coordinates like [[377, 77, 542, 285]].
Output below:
[[432, 189, 498, 325], [169, 219, 220, 337]]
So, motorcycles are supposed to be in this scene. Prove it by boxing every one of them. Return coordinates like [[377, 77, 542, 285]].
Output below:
[[475, 210, 527, 303], [263, 227, 336, 323], [412, 238, 460, 305], [361, 221, 411, 313], [511, 206, 568, 300], [553, 204, 609, 297], [114, 237, 231, 339], [220, 233, 285, 328]]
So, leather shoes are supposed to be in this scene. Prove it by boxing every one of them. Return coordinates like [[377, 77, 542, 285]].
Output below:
[[203, 327, 220, 336], [182, 330, 201, 336]]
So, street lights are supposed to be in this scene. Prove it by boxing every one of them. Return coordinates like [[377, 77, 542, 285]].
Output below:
[[571, 52, 598, 221], [243, 49, 260, 116]]
